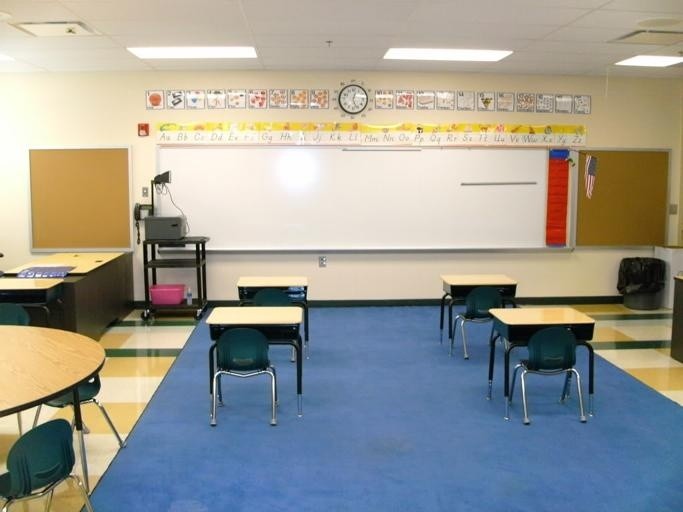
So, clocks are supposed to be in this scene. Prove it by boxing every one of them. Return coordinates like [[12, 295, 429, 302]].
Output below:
[[338, 84, 368, 114]]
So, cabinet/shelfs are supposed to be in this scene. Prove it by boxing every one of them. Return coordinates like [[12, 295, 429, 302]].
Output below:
[[140, 236, 210, 321], [670, 276, 683, 365]]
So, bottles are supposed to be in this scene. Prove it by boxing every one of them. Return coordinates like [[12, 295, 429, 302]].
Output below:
[[186, 286, 193, 305]]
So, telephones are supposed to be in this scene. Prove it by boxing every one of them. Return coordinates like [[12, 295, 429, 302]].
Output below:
[[134, 203, 152, 221]]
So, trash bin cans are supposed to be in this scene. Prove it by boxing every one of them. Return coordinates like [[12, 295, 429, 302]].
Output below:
[[617, 257, 665, 310]]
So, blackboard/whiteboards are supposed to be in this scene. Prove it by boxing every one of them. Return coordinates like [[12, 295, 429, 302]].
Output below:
[[154, 142, 575, 253]]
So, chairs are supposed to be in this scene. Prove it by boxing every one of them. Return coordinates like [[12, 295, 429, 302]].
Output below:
[[0, 301, 127, 512], [508, 326, 586, 425], [451, 286, 508, 360], [252, 288, 292, 306], [210, 327, 280, 427]]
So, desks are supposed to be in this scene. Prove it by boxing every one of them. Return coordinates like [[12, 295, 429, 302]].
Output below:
[[440, 274, 518, 359], [205, 306, 305, 421], [0, 250, 134, 342], [237, 275, 309, 360], [486, 307, 596, 422]]
